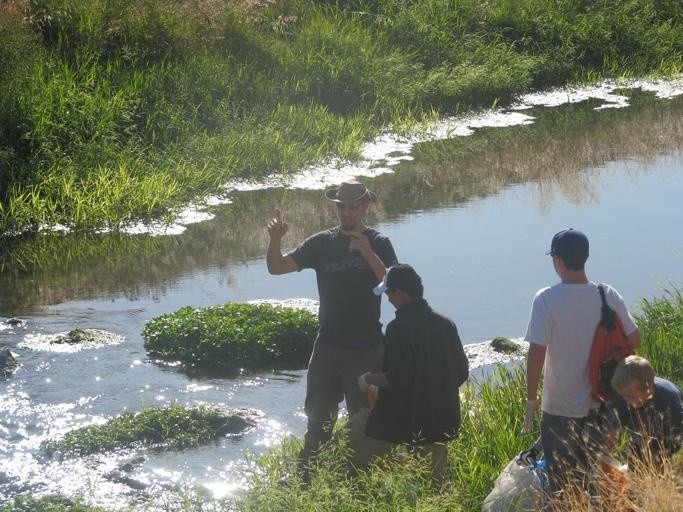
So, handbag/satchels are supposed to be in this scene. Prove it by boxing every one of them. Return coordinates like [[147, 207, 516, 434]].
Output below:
[[588, 311, 637, 402], [482, 448, 545, 512]]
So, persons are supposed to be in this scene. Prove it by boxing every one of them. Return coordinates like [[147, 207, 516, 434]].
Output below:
[[350, 262, 470, 492], [522, 228, 641, 512], [265, 180, 399, 490], [601, 353, 682, 465]]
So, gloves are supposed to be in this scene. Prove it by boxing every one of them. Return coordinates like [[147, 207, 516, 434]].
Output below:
[[357, 371, 378, 394], [520, 396, 540, 434]]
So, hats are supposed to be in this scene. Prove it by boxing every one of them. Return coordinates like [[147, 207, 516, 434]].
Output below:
[[373, 264, 418, 296], [323, 182, 377, 205], [544, 228, 589, 261]]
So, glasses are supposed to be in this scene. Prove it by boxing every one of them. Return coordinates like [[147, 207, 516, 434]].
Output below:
[[335, 202, 362, 210]]
